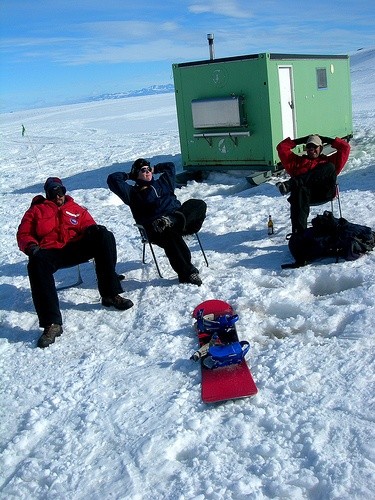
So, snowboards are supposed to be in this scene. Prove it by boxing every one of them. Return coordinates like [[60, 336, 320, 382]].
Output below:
[[192, 299, 259, 404]]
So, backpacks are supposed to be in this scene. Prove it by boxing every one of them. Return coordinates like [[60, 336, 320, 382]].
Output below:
[[281, 211, 375, 268]]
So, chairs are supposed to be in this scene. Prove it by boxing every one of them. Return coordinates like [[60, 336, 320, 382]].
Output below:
[[133, 223, 209, 278]]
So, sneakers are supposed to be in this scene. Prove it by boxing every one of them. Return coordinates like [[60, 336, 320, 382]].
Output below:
[[276, 181, 291, 196], [102, 295, 133, 310], [152, 216, 172, 233], [179, 273, 202, 286], [37, 323, 63, 347]]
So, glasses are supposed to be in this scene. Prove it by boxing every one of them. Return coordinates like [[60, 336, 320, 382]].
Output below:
[[140, 167, 152, 173], [48, 187, 64, 200]]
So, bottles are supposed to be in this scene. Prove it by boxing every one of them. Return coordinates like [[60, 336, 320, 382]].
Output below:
[[209, 334, 222, 349], [268, 216, 273, 235]]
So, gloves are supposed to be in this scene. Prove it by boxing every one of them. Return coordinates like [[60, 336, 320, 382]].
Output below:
[[88, 225, 106, 234], [29, 245, 40, 257]]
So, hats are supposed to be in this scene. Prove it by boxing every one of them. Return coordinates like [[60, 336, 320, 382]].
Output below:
[[44, 177, 64, 192], [306, 135, 322, 146], [131, 159, 150, 177]]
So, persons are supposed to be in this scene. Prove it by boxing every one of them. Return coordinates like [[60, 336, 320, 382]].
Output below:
[[17, 178, 134, 347], [107, 158, 207, 285], [275, 134, 351, 233]]
